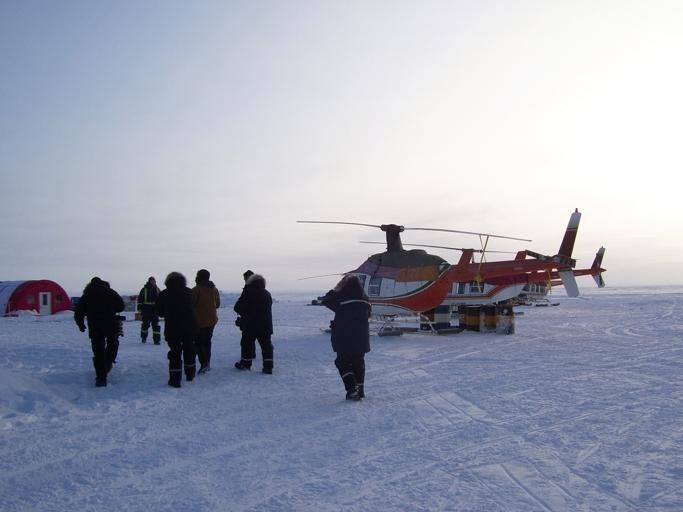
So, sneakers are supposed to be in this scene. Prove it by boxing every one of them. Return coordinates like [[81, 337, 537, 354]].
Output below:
[[235, 362, 248, 370], [346, 385, 362, 400], [198, 363, 209, 374]]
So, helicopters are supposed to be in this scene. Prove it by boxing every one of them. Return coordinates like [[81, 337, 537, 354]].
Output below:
[[514, 279, 564, 308], [356, 235, 610, 309], [296, 208, 584, 338]]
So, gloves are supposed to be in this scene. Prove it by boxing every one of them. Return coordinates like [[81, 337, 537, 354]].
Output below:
[[79, 324, 87, 332]]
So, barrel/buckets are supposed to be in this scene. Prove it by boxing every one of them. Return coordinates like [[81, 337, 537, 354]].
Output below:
[[480, 305, 496, 333], [433, 305, 450, 330], [466, 305, 480, 332], [495, 304, 515, 335], [458, 304, 473, 330], [419, 309, 434, 331]]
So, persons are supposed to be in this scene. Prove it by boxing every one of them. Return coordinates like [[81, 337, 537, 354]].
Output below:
[[192, 269, 220, 374], [322, 276, 371, 400], [243, 269, 256, 358], [74, 276, 126, 387], [136, 276, 161, 345], [154, 271, 197, 387], [234, 274, 273, 374]]
